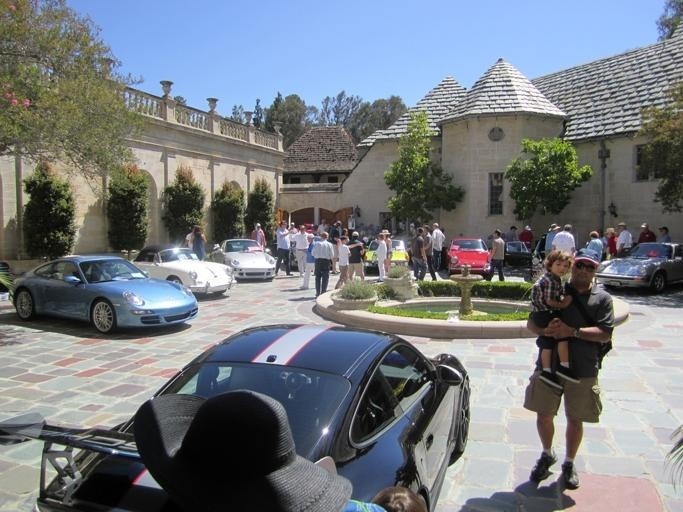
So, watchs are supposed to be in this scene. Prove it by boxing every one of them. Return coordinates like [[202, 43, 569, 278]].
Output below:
[[574, 327, 581, 338]]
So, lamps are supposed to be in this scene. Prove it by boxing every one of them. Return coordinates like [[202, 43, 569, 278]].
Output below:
[[606, 201, 618, 220], [354, 203, 360, 217]]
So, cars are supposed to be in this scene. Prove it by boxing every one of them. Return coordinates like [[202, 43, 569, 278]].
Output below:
[[442, 237, 495, 280], [286, 222, 325, 271], [502, 234, 547, 281], [361, 239, 410, 275]]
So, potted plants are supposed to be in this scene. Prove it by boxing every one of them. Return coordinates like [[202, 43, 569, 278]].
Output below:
[[329, 276, 376, 311], [380, 263, 417, 300]]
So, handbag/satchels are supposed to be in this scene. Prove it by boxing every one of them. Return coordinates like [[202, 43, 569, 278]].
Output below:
[[600, 341, 612, 357]]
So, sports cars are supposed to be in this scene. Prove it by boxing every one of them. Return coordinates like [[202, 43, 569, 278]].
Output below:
[[0, 323, 472, 512], [592, 241, 683, 294], [7, 254, 200, 333], [206, 238, 279, 281], [129, 243, 235, 297]]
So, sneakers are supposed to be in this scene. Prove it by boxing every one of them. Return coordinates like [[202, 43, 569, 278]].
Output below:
[[556, 366, 581, 383], [539, 371, 564, 390], [562, 461, 580, 488], [530, 449, 558, 480]]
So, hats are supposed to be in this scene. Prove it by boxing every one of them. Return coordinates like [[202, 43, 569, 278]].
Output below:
[[380, 229, 391, 234], [549, 224, 560, 231], [605, 227, 618, 236], [575, 248, 599, 266], [659, 227, 668, 232]]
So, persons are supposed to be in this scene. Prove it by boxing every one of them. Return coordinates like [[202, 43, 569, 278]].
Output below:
[[523, 248, 614, 489], [370, 486, 428, 512], [185, 225, 207, 260], [251, 220, 446, 299], [487, 220, 671, 281], [530, 250, 580, 389]]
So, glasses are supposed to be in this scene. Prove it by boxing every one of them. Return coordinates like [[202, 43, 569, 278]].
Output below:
[[575, 262, 595, 272]]
